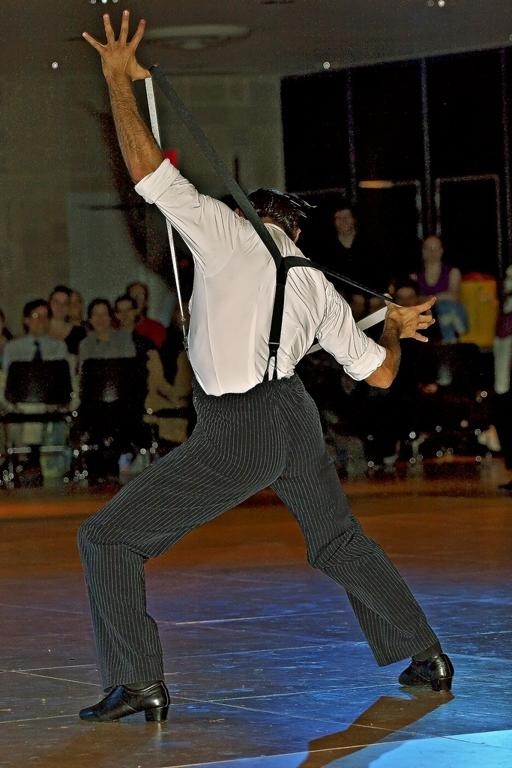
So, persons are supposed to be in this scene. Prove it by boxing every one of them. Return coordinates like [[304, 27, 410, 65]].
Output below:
[[294, 203, 512, 472], [77, 10, 454, 722], [0, 282, 198, 488]]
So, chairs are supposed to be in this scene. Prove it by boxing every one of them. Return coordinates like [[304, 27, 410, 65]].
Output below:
[[0, 360, 73, 487]]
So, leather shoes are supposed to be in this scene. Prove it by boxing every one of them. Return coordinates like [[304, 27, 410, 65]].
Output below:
[[79, 682, 171, 725], [398, 653, 455, 692]]
